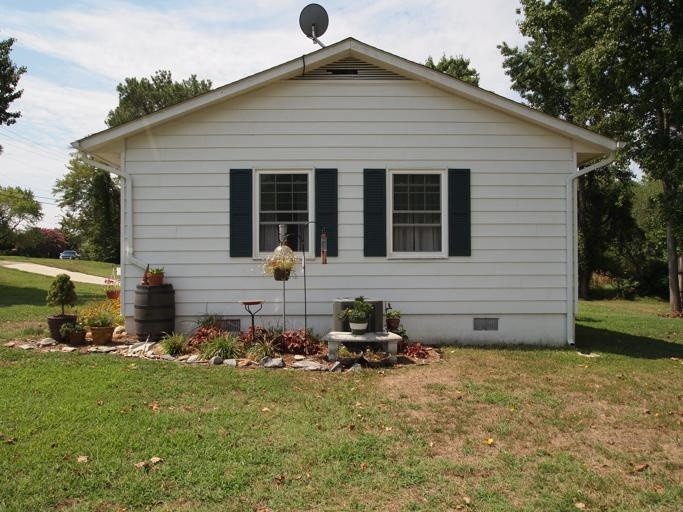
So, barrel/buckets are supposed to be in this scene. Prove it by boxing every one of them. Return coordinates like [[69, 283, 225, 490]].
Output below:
[[134, 284, 175, 341]]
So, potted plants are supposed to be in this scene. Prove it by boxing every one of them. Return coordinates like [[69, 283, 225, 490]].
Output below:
[[45, 273, 117, 344], [261, 245, 300, 281], [145, 265, 165, 286], [333, 296, 404, 367], [103, 276, 121, 299]]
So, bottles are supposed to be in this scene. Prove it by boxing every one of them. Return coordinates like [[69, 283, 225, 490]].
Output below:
[[279, 223, 287, 246], [320, 227, 327, 264]]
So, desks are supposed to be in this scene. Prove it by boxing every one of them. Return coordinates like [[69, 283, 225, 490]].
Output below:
[[320, 331, 403, 361]]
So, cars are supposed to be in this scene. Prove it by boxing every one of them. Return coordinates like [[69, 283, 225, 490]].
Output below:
[[60, 250, 81, 260]]
